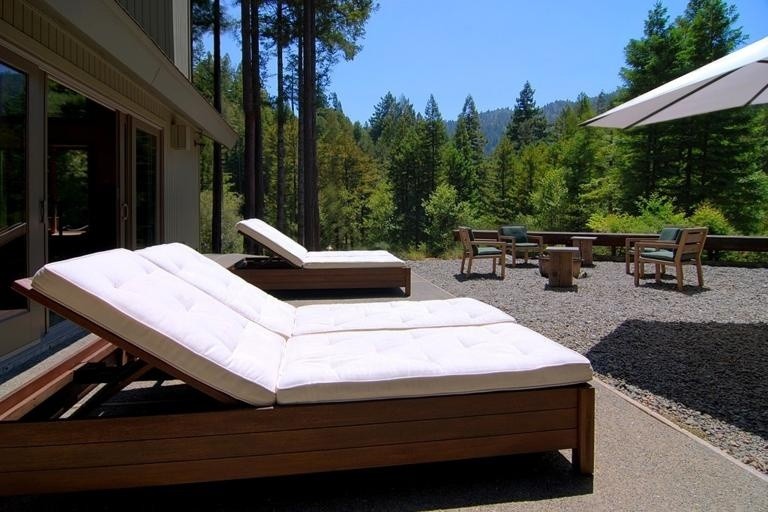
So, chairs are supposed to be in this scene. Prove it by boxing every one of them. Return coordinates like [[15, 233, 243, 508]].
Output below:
[[457, 226, 506, 279], [625, 223, 689, 278], [635, 226, 708, 292], [134, 242, 517, 341], [226, 217, 411, 297], [0, 246, 595, 497], [497, 224, 543, 268]]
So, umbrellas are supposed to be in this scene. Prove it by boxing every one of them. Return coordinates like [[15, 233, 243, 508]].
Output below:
[[576, 31, 768, 129]]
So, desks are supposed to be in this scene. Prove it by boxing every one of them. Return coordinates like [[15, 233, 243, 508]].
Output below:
[[546, 246, 580, 287], [538, 255, 584, 278], [570, 235, 597, 266]]
